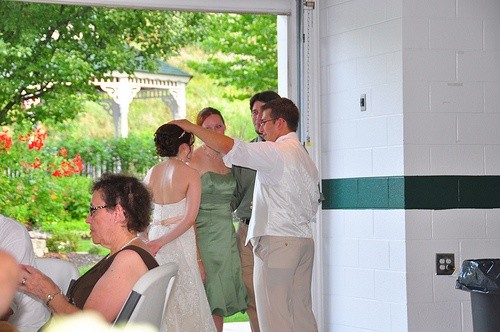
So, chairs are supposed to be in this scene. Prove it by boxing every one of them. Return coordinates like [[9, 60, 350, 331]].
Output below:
[[112, 262, 179, 332]]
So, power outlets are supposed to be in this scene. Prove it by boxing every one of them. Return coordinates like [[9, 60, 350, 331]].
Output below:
[[435, 253, 456, 276]]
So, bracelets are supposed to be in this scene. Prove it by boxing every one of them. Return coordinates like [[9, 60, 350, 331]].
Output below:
[[47, 291, 61, 306], [198, 259, 201, 261]]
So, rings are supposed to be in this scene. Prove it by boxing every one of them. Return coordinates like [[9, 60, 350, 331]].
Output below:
[[22, 279, 26, 285]]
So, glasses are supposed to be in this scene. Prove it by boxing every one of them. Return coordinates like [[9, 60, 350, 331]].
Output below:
[[88, 204, 126, 216], [260, 116, 285, 127]]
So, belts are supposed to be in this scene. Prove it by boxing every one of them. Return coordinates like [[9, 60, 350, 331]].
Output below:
[[241, 218, 250, 225]]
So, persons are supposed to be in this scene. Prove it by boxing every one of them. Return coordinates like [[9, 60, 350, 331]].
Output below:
[[16, 176, 159, 332], [0, 214, 51, 332], [169, 98, 321, 332], [187, 108, 250, 332], [142, 124, 217, 332], [232, 91, 280, 332]]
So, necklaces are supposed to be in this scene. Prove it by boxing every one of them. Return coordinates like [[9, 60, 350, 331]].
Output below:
[[175, 157, 186, 163], [106, 237, 139, 259], [202, 145, 216, 159]]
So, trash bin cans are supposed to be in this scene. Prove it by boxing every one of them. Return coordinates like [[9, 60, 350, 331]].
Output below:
[[464, 259, 500, 332]]
